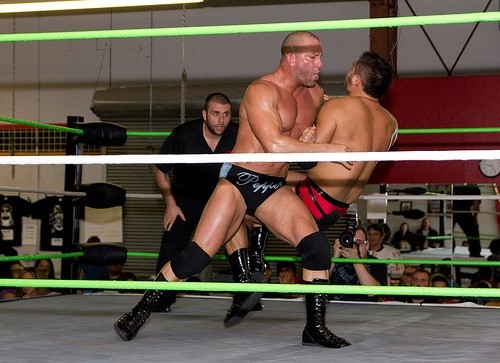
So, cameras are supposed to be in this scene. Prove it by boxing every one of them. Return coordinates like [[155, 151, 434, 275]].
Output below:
[[339, 231, 354, 249]]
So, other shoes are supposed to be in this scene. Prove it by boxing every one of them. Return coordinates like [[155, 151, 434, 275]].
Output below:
[[152, 303, 171, 314], [250, 301, 264, 312]]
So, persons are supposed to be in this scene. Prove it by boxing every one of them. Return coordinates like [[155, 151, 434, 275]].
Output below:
[[116, 30, 357, 348], [268, 184, 500, 304], [223, 52, 401, 327], [0, 235, 140, 301], [150, 93, 253, 313]]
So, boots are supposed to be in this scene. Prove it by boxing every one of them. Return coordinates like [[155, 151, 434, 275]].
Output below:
[[250, 225, 271, 284], [223, 247, 265, 329], [114, 272, 173, 342], [301, 277, 352, 349]]
